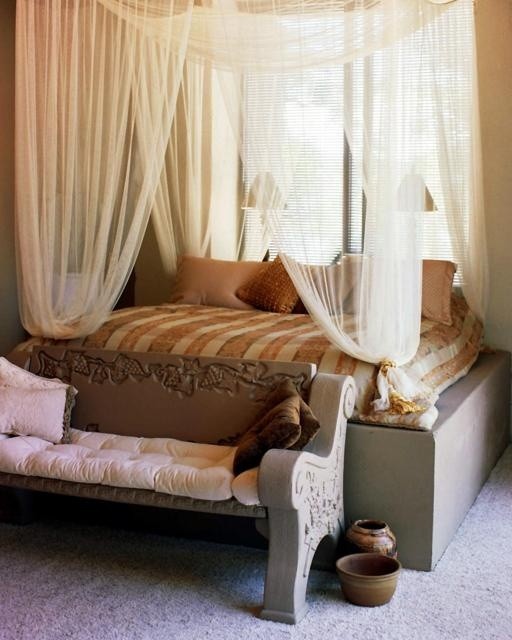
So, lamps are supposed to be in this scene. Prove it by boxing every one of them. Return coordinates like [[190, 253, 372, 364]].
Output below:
[[387, 161, 439, 255], [241, 172, 291, 256]]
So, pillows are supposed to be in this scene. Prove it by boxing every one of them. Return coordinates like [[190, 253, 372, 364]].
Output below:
[[233, 253, 308, 315], [301, 262, 359, 315], [2, 381, 76, 448], [170, 254, 273, 310], [232, 395, 303, 480], [252, 378, 322, 454], [339, 256, 458, 327], [1, 358, 80, 400]]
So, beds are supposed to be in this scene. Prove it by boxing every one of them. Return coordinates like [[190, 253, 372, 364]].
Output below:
[[5, 252, 512, 573]]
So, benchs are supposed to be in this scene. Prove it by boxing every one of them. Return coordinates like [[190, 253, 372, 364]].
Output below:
[[1, 345, 360, 625]]
